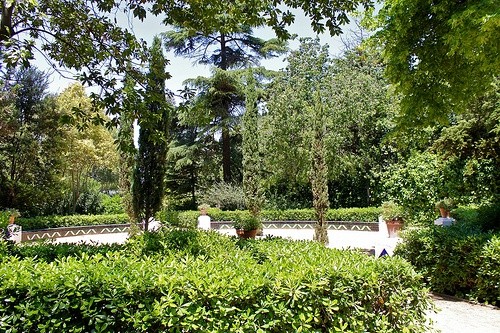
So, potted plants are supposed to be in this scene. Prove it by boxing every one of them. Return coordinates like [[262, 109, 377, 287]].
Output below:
[[380, 199, 405, 239], [435, 200, 452, 219], [198, 203, 211, 215], [3, 207, 21, 225], [231, 212, 260, 240]]
